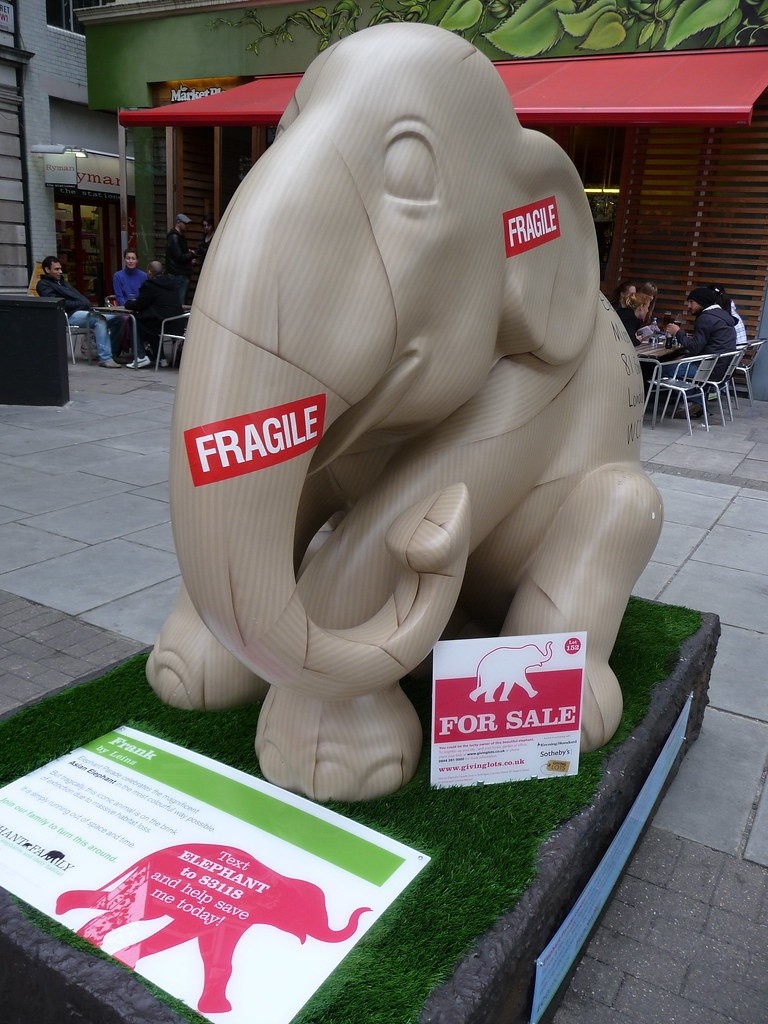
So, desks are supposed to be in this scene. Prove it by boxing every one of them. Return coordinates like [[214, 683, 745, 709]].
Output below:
[[86, 306, 138, 371], [634, 343, 680, 358]]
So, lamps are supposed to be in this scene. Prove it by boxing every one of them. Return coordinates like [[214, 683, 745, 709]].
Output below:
[[29, 144, 88, 158]]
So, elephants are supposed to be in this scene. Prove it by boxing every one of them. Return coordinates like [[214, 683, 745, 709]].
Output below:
[[143, 21, 666, 804]]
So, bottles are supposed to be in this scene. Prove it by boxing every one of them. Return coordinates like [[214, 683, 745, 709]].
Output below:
[[666, 332, 672, 348], [650, 318, 657, 337]]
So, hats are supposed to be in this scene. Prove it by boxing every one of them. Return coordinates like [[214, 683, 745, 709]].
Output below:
[[176, 214, 192, 223], [686, 288, 717, 309]]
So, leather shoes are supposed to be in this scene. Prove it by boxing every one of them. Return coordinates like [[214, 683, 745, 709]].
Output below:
[[98, 358, 122, 368], [114, 356, 127, 363]]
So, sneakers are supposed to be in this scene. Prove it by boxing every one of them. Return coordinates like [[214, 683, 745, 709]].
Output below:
[[125, 355, 150, 368], [690, 410, 700, 420], [674, 401, 701, 418], [160, 358, 169, 367]]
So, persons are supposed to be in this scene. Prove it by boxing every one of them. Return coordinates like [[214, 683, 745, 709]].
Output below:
[[36, 213, 215, 369], [609, 279, 748, 419]]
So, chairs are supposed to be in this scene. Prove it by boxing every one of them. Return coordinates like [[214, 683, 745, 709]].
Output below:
[[104, 295, 117, 306], [154, 312, 190, 372], [65, 310, 96, 365], [639, 338, 768, 436]]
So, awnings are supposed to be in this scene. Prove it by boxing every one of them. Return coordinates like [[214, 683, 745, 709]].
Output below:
[[119, 46, 768, 128]]
